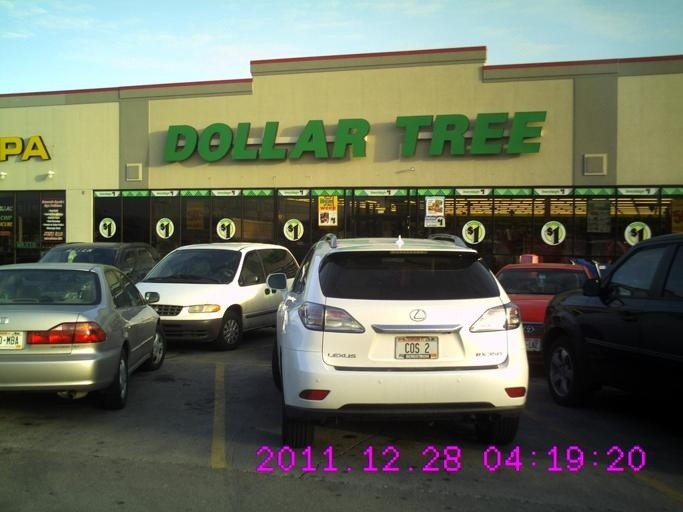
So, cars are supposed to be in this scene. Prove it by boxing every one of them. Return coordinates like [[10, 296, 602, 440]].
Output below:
[[493, 264, 590, 360], [422, 219, 629, 284], [265, 233, 529, 450], [0, 262, 166, 411], [36, 242, 162, 284]]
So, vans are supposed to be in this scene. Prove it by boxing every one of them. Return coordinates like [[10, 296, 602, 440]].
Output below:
[[133, 241, 300, 350]]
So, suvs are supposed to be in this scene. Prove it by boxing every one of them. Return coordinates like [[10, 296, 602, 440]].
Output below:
[[540, 232, 681, 406]]
